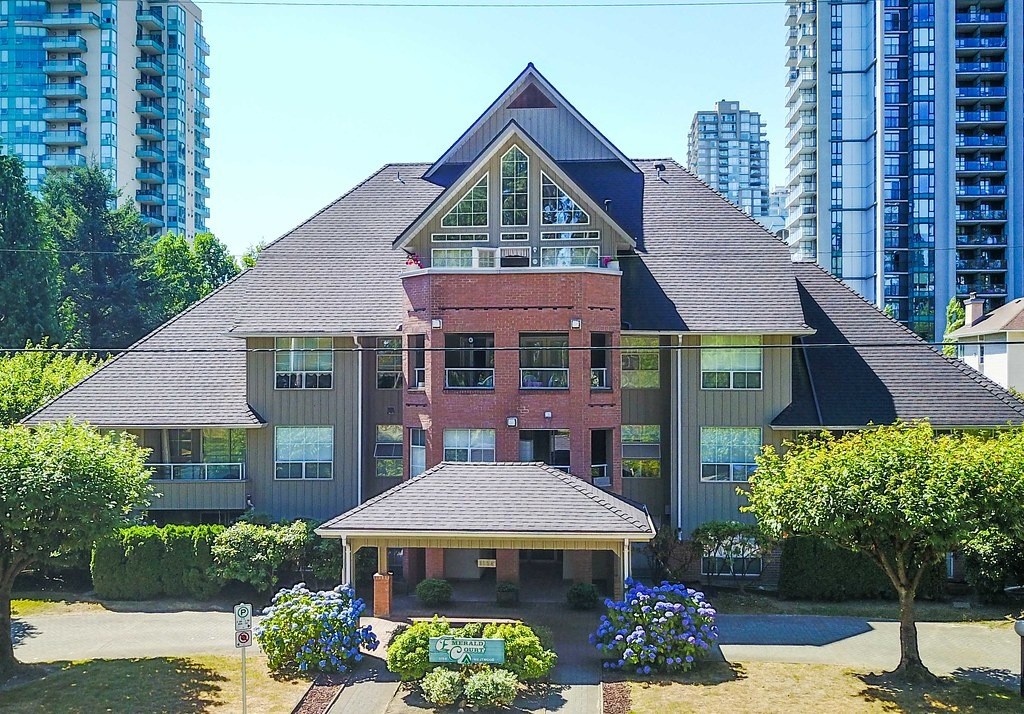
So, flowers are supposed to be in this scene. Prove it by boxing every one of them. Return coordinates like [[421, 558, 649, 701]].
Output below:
[[405, 255, 424, 268], [253, 583, 379, 679], [588, 577, 719, 675], [603, 256, 618, 266]]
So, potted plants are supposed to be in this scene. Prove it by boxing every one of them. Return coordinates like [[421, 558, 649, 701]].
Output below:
[[567, 581, 600, 609], [497, 579, 522, 606], [415, 578, 456, 608]]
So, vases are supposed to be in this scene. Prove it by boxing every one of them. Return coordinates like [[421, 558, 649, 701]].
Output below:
[[607, 260, 619, 270], [407, 264, 420, 270]]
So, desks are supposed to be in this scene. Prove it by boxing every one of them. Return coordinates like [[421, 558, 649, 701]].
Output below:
[[407, 617, 518, 626]]
